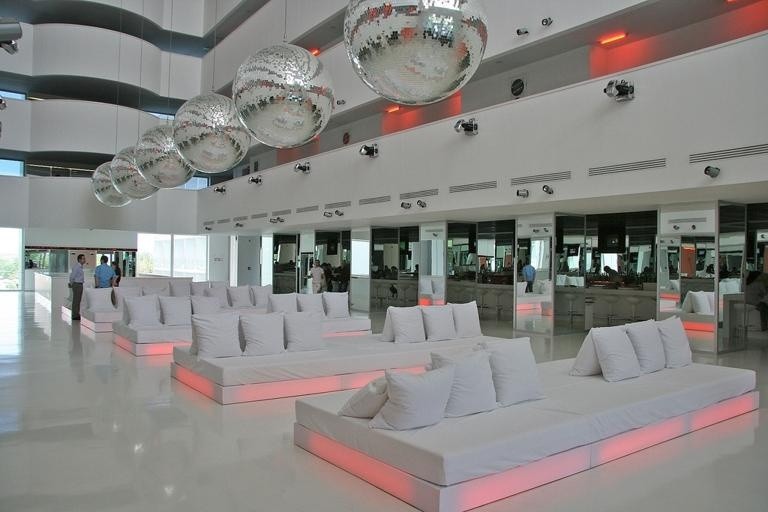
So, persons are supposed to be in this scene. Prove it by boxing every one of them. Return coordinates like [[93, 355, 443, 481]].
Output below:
[[94, 256, 116, 288], [522, 264, 536, 292], [371, 264, 418, 280], [111, 261, 121, 287], [603, 266, 623, 282], [273, 260, 350, 294], [68, 254, 85, 320]]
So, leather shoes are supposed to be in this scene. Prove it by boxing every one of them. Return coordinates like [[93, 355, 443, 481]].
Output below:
[[72, 317, 81, 320]]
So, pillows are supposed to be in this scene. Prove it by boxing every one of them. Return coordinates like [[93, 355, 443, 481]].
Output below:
[[380, 300, 483, 345], [336, 336, 548, 431], [568, 315, 692, 382], [80, 279, 373, 363]]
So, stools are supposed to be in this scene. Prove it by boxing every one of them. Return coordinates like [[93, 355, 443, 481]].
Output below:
[[399, 282, 418, 305], [478, 289, 514, 315], [602, 296, 656, 326]]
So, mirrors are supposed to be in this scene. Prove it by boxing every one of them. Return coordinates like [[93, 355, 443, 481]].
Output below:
[[418, 220, 479, 307], [657, 200, 748, 354], [514, 212, 588, 337], [349, 226, 401, 311]]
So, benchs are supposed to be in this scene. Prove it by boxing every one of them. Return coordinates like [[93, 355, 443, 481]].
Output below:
[[171, 335, 510, 405], [294, 358, 759, 512]]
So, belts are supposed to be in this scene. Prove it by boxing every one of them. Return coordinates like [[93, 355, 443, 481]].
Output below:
[[72, 282, 83, 284]]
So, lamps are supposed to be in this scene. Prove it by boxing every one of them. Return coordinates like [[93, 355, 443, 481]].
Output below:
[[704, 165, 720, 178]]
